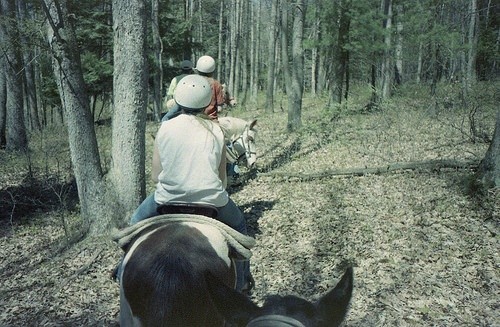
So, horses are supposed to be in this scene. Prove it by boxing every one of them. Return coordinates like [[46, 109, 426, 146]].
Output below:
[[214, 116, 260, 172], [112, 201, 237, 327], [202, 261, 355, 327]]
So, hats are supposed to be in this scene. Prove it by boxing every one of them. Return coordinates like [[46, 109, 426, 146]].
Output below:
[[181, 60, 192, 69]]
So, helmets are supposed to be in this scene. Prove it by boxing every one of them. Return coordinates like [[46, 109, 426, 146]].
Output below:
[[173, 74, 212, 108], [196, 56, 216, 73]]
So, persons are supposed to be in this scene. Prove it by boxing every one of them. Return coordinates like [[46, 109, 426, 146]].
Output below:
[[166, 60, 196, 112], [109, 74, 253, 297], [161, 103, 182, 122], [195, 55, 225, 122]]
